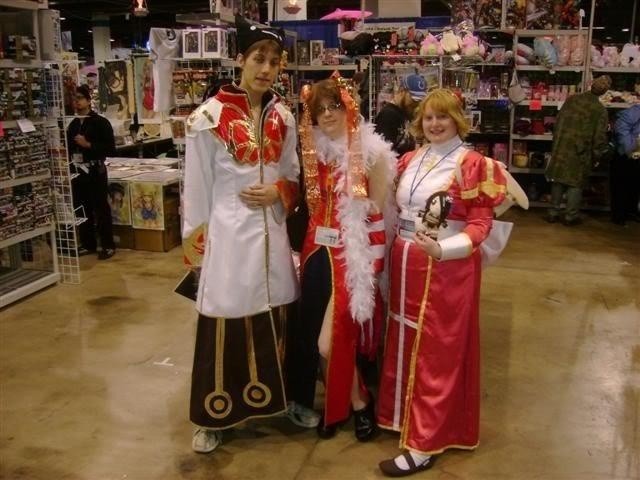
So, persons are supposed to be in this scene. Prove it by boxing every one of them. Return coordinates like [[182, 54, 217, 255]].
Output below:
[[67, 84, 117, 259], [367, 88, 508, 471], [543, 74, 612, 225], [612, 81, 640, 225], [417, 191, 452, 241], [180, 12, 321, 454], [280, 70, 401, 442]]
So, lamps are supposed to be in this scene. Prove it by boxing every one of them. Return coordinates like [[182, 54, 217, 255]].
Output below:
[[133, 0, 150, 17], [283, 0, 301, 14]]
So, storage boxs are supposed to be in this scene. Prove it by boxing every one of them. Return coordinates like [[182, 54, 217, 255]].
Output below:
[[109, 197, 180, 252]]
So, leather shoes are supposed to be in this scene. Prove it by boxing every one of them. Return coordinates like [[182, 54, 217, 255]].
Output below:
[[270, 387, 376, 442], [542, 212, 582, 225]]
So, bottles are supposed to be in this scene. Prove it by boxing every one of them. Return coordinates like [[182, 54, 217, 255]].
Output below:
[[297, 40, 310, 65], [547, 85, 577, 101], [489, 79, 499, 100], [500, 73, 509, 97], [443, 70, 480, 93]]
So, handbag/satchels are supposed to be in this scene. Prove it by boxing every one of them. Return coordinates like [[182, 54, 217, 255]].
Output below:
[[507, 70, 527, 104]]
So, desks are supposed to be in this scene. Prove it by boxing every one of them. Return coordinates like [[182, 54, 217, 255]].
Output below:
[[115, 137, 174, 158]]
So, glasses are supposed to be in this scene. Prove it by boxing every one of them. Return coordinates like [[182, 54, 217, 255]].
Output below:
[[314, 102, 343, 113], [76, 95, 86, 99]]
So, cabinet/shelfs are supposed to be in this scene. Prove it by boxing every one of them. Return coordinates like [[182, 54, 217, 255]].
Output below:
[[0, 59, 60, 309], [439, 30, 640, 208]]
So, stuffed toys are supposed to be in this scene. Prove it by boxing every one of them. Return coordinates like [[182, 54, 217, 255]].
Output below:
[[419, 25, 485, 58], [515, 31, 640, 105]]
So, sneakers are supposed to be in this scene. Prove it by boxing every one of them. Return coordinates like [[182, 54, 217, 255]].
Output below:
[[379, 450, 435, 477], [192, 425, 223, 453], [70, 246, 96, 256], [97, 248, 115, 259]]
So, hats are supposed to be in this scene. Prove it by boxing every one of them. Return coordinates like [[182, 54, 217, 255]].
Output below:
[[591, 75, 612, 96], [235, 13, 285, 53], [399, 73, 427, 102]]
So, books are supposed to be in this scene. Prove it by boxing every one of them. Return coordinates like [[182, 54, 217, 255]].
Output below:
[[175, 271, 197, 301]]
